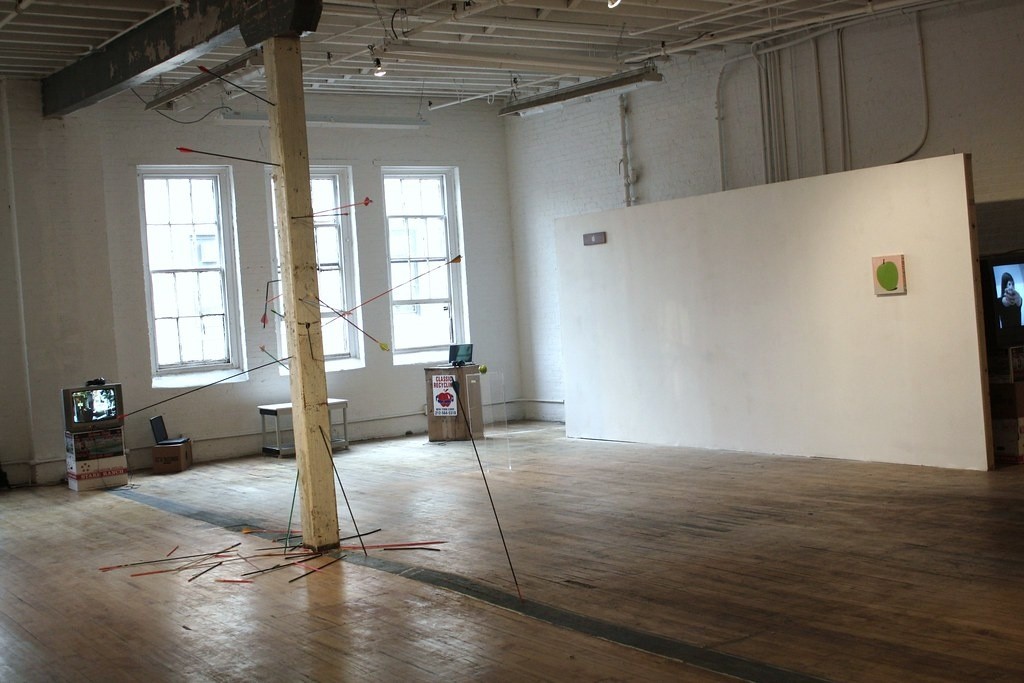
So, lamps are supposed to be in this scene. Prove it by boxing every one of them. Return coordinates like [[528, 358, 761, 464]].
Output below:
[[497, 41, 666, 117], [374, 58, 387, 78], [145, 44, 265, 111]]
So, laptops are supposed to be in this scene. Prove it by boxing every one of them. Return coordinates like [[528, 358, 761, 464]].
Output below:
[[151, 415, 190, 445], [438, 344, 473, 367]]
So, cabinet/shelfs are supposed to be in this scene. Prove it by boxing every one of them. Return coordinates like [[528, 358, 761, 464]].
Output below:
[[257, 397, 350, 457]]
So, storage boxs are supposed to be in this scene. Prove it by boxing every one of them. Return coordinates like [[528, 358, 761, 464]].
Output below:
[[152, 440, 193, 473], [66, 454, 129, 491], [63, 426, 125, 460], [990, 381, 1024, 465]]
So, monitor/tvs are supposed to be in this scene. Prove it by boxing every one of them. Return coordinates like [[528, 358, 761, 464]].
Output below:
[[980, 251, 1024, 348], [62, 384, 124, 432]]
